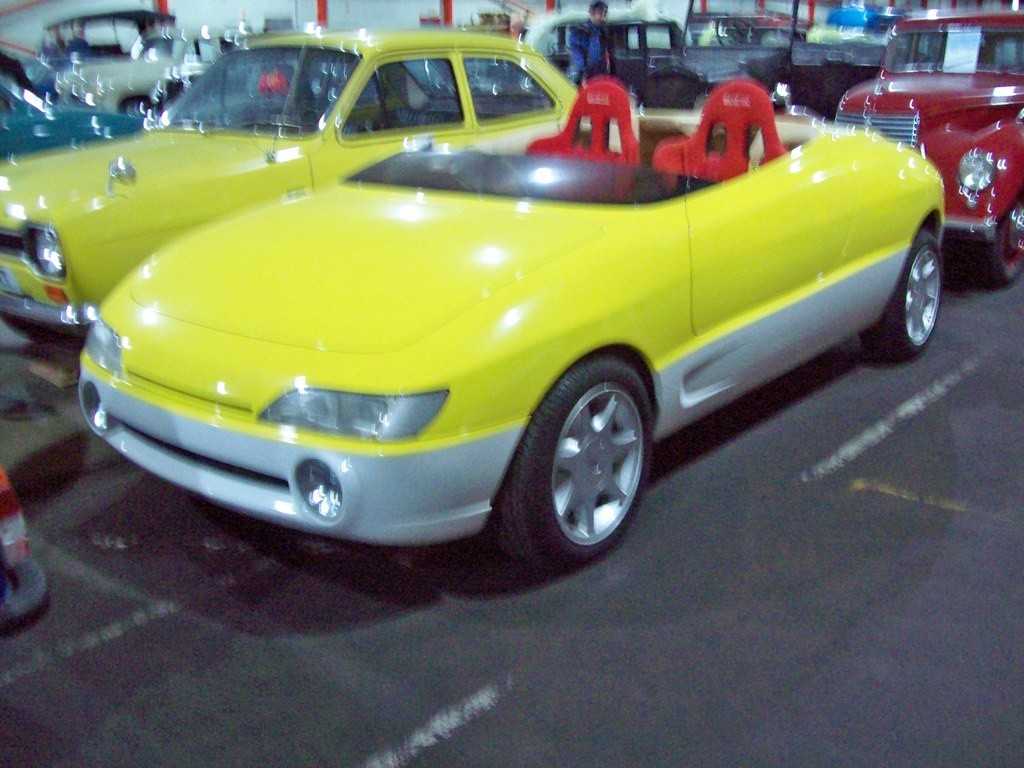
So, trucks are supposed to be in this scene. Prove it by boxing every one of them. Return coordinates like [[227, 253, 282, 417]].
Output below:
[[678, 0, 929, 125]]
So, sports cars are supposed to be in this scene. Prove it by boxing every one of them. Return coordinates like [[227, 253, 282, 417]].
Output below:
[[73, 80, 945, 567]]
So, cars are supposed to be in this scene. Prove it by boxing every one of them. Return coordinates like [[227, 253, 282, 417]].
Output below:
[[0, 11, 238, 162], [839, 8, 1022, 284], [0, 31, 578, 354]]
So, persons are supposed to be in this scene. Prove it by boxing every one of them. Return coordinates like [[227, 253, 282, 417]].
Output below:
[[65, 28, 91, 67], [563, 0, 618, 86]]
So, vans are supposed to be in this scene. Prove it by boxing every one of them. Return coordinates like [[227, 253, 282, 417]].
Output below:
[[542, 20, 684, 86]]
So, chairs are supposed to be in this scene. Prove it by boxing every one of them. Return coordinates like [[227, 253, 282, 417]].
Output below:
[[527, 74, 642, 206], [651, 81, 787, 196]]
